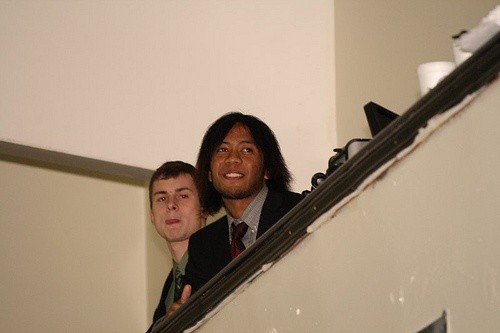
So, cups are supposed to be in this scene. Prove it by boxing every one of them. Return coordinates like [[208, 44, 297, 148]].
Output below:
[[418, 62, 455, 99]]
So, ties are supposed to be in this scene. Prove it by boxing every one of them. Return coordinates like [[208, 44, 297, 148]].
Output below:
[[230, 222, 248, 260], [173, 267, 184, 303]]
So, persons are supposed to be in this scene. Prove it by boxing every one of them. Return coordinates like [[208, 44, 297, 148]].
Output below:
[[149, 111, 304, 333], [149, 161, 211, 321]]
